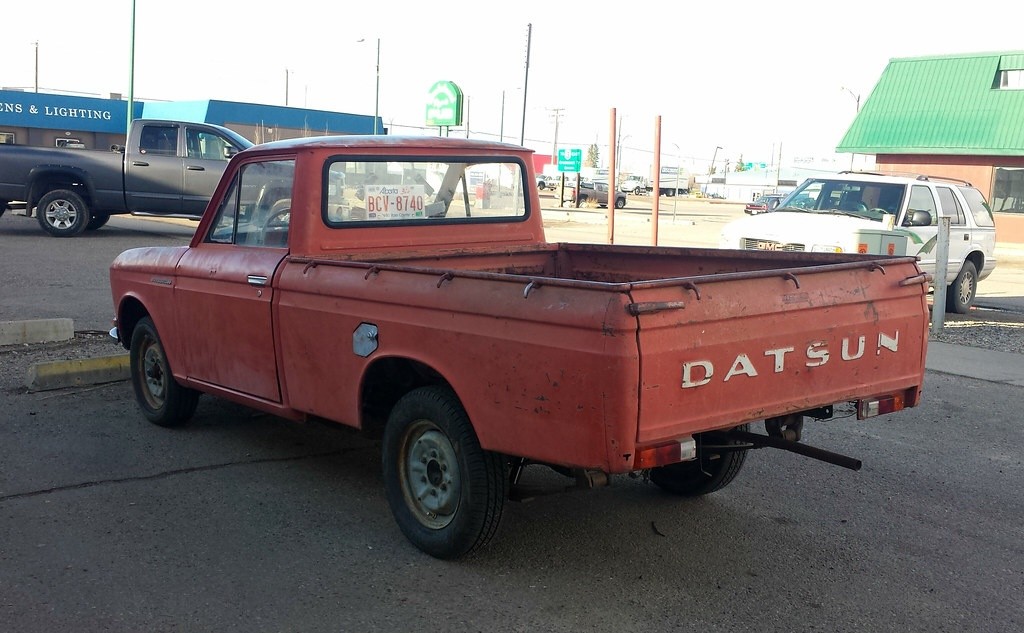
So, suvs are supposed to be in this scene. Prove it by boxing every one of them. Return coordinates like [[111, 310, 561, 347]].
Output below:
[[744, 194, 785, 216]]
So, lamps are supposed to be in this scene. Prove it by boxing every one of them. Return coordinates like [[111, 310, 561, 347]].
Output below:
[[266, 128, 272, 134]]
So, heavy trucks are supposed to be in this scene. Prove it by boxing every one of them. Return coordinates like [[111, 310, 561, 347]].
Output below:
[[621, 174, 689, 197]]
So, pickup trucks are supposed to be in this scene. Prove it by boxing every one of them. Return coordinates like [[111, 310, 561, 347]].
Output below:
[[108, 135, 930, 559], [535, 174, 587, 190], [0, 119, 351, 237], [554, 182, 626, 209], [726, 170, 997, 315]]
